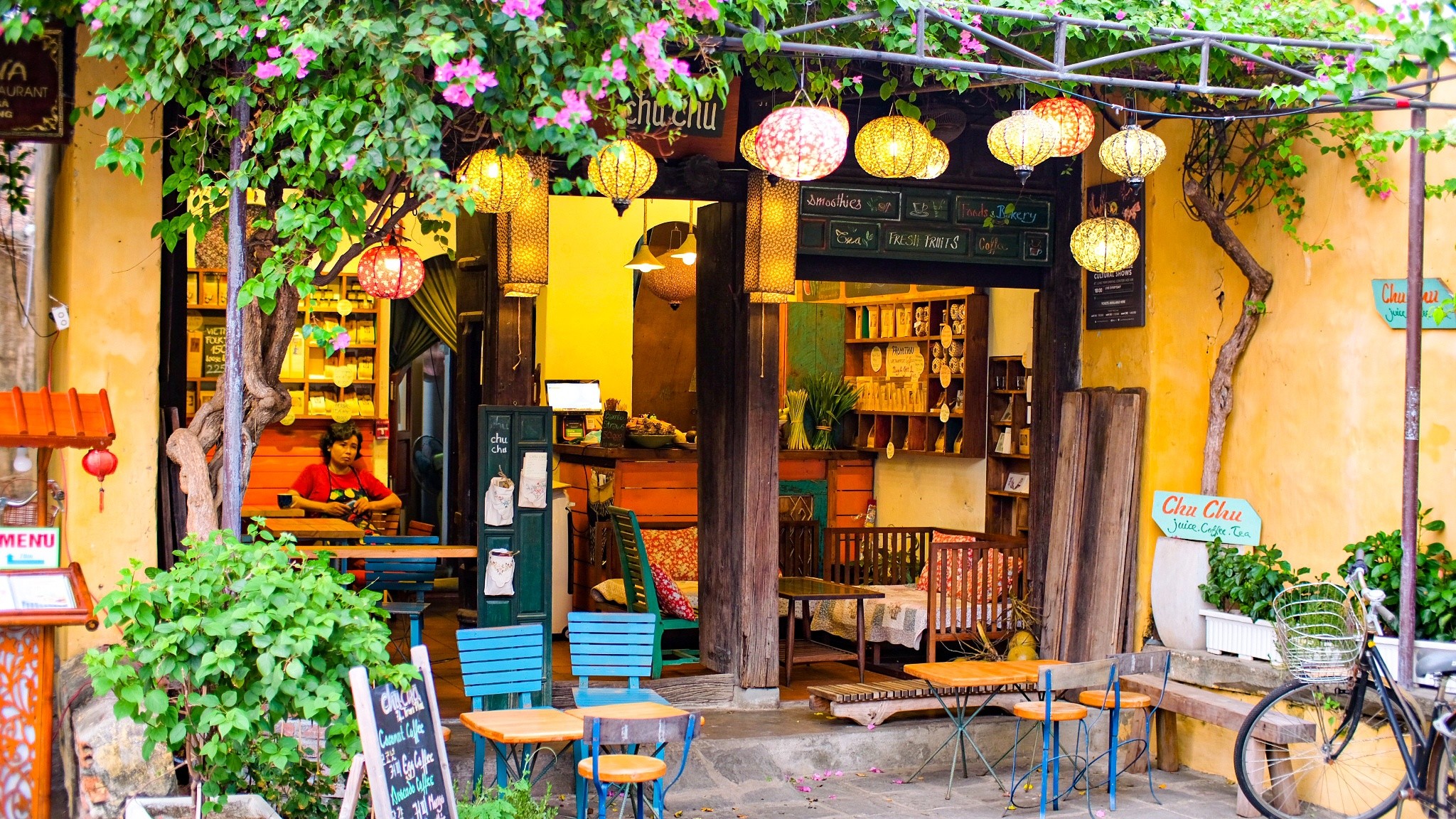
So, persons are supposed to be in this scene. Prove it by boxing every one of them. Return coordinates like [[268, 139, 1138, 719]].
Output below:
[[287, 419, 402, 570]]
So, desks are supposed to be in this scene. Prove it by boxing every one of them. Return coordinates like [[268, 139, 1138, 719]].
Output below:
[[776, 576, 886, 689], [902, 659, 1072, 801], [561, 704, 704, 818], [238, 507, 365, 596], [459, 708, 585, 788]]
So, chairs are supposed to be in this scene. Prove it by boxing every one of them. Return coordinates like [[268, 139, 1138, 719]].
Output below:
[[336, 507, 440, 648], [606, 505, 700, 679], [455, 611, 696, 819]]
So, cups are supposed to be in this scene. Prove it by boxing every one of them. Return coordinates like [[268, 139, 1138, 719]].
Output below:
[[277, 494, 293, 510], [996, 376, 1006, 389], [1013, 376, 1025, 390]]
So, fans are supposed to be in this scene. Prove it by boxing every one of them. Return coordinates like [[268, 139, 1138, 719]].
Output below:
[[413, 434, 444, 498]]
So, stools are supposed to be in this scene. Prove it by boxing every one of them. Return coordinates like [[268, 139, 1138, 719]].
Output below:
[[1002, 650, 1173, 819]]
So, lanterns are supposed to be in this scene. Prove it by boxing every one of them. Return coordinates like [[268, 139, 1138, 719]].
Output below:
[[645, 249, 697, 305], [987, 110, 1058, 176], [743, 172, 801, 306], [83, 450, 118, 477], [356, 244, 425, 302], [854, 117, 932, 178], [1098, 125, 1167, 181], [1029, 97, 1096, 158], [1069, 218, 1141, 271], [457, 149, 533, 214], [912, 138, 949, 179], [587, 140, 656, 201], [494, 157, 550, 295], [739, 105, 848, 181]]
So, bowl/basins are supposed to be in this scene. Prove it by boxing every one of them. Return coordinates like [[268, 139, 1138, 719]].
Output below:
[[628, 433, 677, 448]]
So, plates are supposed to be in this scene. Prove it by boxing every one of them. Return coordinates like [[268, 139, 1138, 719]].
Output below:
[[676, 442, 697, 449]]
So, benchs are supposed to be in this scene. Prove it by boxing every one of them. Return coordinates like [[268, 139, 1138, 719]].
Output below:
[[594, 520, 818, 620], [809, 681, 1057, 727], [819, 527, 1027, 665], [1114, 673, 1316, 819]]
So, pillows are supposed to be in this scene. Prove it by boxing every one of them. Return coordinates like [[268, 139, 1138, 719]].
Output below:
[[633, 527, 699, 623], [916, 531, 1025, 604]]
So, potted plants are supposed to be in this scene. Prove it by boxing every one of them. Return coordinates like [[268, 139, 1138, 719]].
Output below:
[[1197, 498, 1456, 688], [81, 517, 425, 819]]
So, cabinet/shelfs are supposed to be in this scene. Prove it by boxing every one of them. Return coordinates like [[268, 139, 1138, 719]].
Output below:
[[984, 353, 1033, 540], [185, 265, 389, 425], [840, 292, 986, 458]]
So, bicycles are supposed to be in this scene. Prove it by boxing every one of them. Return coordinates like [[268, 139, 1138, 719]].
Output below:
[[1232, 547, 1456, 819]]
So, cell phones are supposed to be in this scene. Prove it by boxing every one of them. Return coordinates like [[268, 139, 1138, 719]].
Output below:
[[345, 500, 357, 511]]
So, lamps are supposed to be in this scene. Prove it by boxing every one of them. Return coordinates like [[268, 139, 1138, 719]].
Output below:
[[502, 153, 552, 301], [623, 199, 665, 273], [670, 200, 696, 266]]
